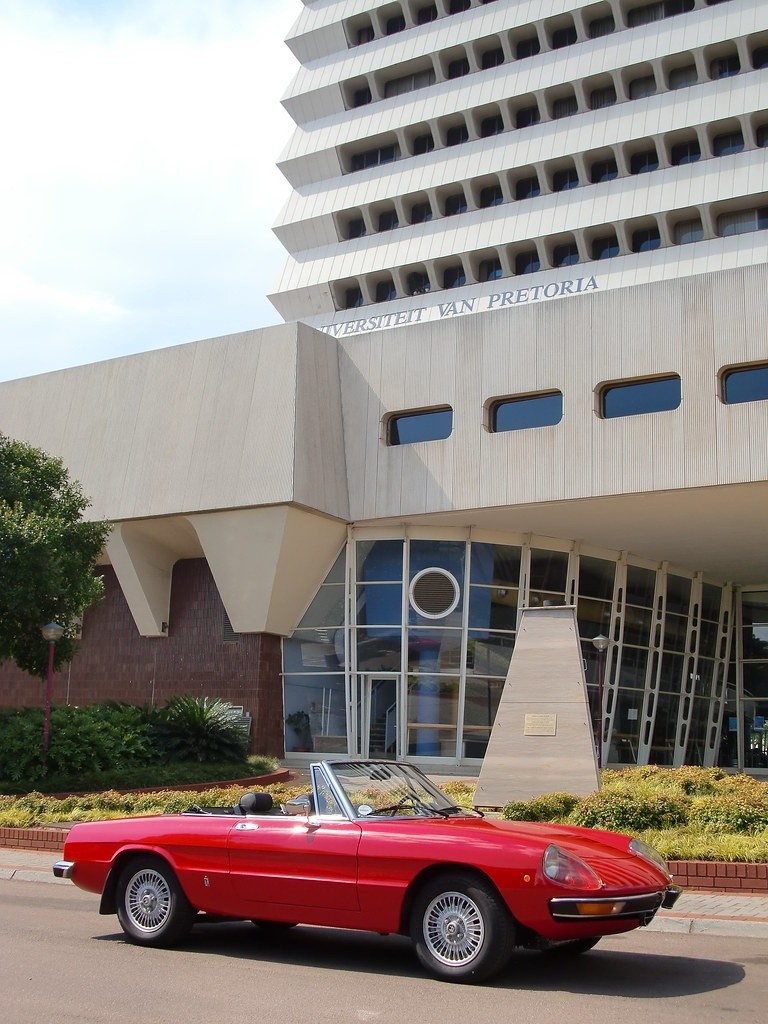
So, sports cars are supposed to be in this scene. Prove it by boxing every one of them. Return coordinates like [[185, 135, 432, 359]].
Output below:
[[53, 760, 682, 984]]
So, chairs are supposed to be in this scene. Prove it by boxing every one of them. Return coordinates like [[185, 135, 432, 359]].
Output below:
[[240, 792, 278, 816], [296, 793, 328, 814]]
